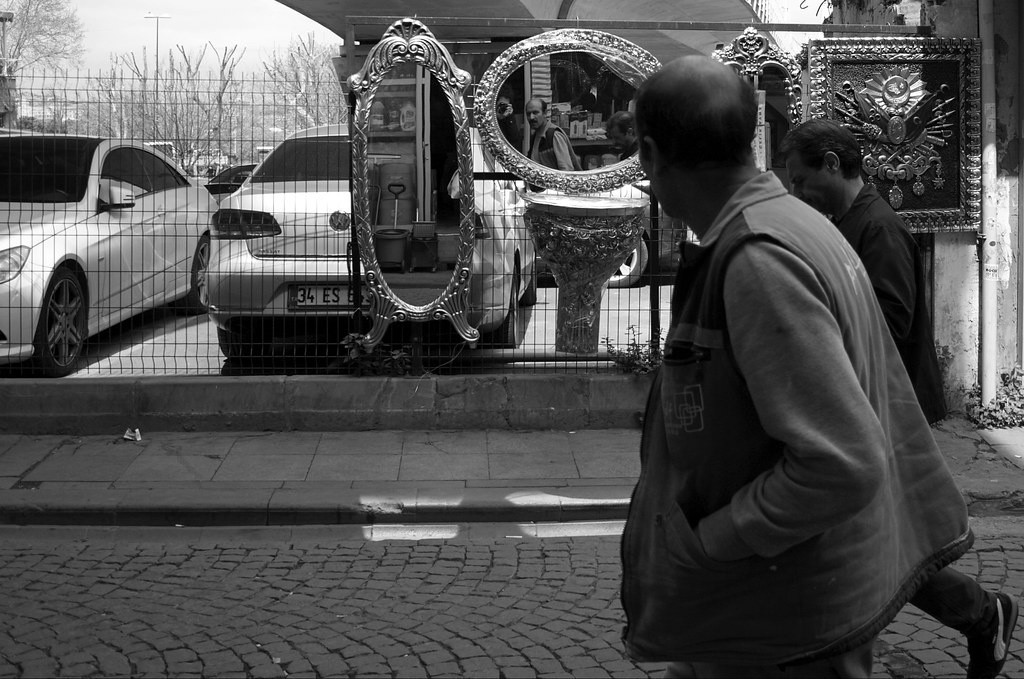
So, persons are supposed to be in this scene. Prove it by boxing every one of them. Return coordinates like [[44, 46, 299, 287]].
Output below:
[[620, 53, 974, 679], [780, 116, 1018, 679], [607, 110, 640, 161], [525, 98, 583, 171], [496, 82, 522, 152], [207, 166, 213, 177]]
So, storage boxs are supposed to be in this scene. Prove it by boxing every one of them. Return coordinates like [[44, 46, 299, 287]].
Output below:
[[583, 154, 600, 170], [552, 115, 560, 127], [551, 102, 572, 112], [593, 122, 600, 128], [569, 115, 578, 140], [560, 114, 569, 129], [579, 111, 588, 139], [601, 153, 619, 167], [412, 220, 437, 238], [600, 122, 607, 127], [594, 113, 602, 123], [587, 113, 593, 126]]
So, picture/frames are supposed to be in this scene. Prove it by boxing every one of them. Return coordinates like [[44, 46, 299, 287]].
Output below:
[[807, 36, 981, 235]]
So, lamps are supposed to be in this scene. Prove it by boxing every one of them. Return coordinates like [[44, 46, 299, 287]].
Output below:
[[341, 17, 484, 355]]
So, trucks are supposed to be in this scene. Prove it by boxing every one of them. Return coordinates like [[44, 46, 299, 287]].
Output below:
[[277, 0, 784, 293]]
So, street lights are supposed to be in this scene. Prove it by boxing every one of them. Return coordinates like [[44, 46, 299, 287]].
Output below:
[[143, 15, 172, 101], [0, 12, 15, 58]]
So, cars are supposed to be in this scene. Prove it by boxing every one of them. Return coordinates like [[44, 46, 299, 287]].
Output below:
[[0, 133, 220, 377], [202, 160, 257, 202], [204, 125, 539, 363], [184, 148, 230, 180]]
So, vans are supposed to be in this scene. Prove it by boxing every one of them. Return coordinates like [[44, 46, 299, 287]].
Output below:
[[142, 142, 177, 163]]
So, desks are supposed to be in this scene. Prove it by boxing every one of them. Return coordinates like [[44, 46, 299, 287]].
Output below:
[[570, 138, 615, 146]]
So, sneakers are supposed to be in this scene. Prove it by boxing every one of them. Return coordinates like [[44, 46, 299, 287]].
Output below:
[[968, 592, 1018, 679]]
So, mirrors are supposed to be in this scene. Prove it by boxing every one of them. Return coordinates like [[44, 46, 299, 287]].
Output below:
[[714, 26, 804, 198], [472, 27, 661, 194]]
